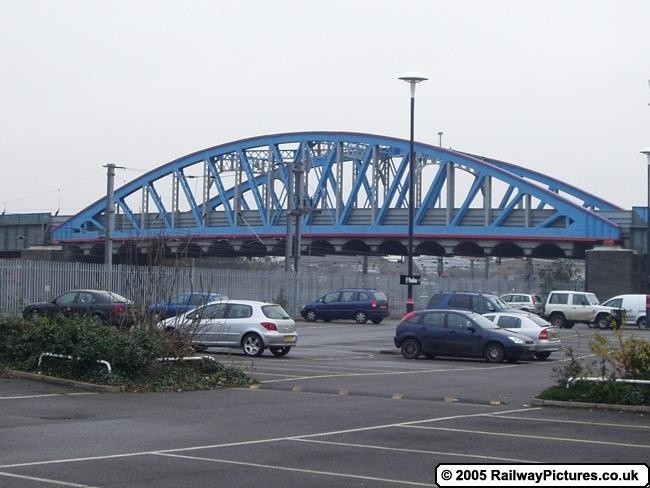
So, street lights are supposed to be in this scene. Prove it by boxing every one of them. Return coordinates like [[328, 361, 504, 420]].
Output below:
[[639, 149, 650, 292], [397, 72, 430, 313], [438, 131, 443, 208]]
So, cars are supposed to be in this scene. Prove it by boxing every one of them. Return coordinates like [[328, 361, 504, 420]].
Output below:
[[394, 290, 650, 363], [23, 289, 298, 356], [300, 288, 390, 324]]
